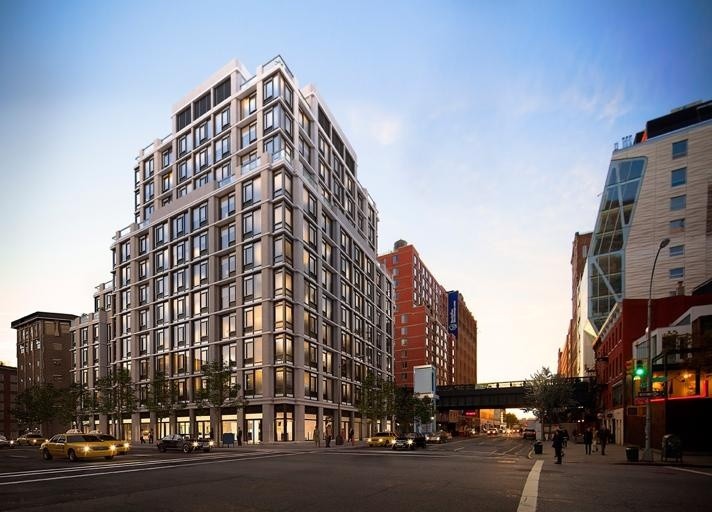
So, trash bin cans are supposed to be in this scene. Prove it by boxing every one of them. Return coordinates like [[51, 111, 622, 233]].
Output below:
[[625, 447, 639, 462], [336, 435, 344, 445], [533, 442, 543, 454], [662, 434, 684, 461]]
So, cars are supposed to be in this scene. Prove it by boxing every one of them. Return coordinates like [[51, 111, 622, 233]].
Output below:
[[486, 427, 536, 440], [157, 433, 213, 454], [367, 430, 453, 451], [0, 429, 129, 462]]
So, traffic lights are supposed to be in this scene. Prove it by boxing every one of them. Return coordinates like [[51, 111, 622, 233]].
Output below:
[[636, 359, 644, 378]]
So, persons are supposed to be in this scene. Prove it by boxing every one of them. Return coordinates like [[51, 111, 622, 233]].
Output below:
[[140, 428, 146, 444], [313, 424, 322, 448], [209, 427, 215, 440], [552, 425, 610, 464], [324, 424, 333, 448], [237, 427, 243, 446], [347, 425, 354, 443], [148, 428, 154, 444]]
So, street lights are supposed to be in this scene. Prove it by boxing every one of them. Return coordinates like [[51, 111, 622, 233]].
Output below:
[[640, 237, 671, 462], [336, 354, 366, 445]]
